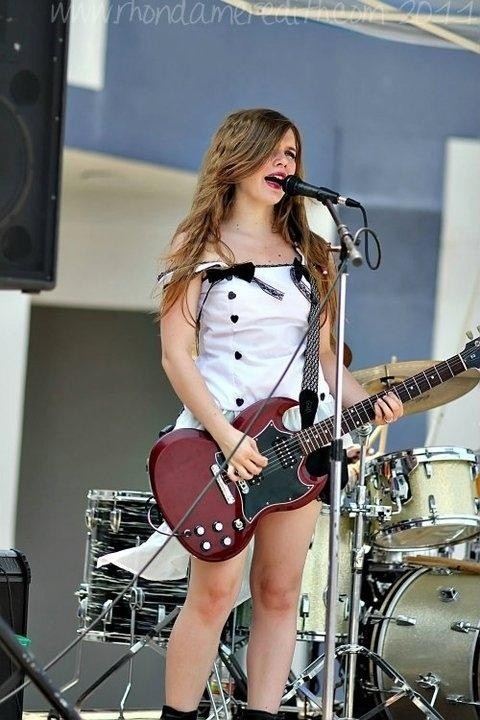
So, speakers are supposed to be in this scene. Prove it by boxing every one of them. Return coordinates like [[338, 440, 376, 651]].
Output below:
[[0, 1, 72, 295], [0, 546, 32, 719]]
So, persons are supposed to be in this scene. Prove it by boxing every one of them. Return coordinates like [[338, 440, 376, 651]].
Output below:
[[159, 109, 404, 719]]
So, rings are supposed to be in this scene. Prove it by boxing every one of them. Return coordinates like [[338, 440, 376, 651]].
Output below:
[[384, 417, 392, 425]]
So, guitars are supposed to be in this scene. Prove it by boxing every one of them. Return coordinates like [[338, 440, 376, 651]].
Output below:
[[150, 323, 480, 561]]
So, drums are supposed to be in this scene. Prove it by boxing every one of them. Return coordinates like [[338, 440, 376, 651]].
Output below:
[[234, 503, 358, 642], [360, 446, 480, 551], [81, 489, 228, 646], [368, 565, 480, 719]]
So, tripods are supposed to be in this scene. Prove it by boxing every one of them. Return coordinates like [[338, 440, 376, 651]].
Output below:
[[279, 441, 443, 719]]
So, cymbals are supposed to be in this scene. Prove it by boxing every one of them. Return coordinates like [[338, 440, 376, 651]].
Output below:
[[345, 361, 480, 416]]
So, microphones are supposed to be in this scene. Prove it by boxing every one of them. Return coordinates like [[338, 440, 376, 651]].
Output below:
[[278, 172, 362, 213]]
